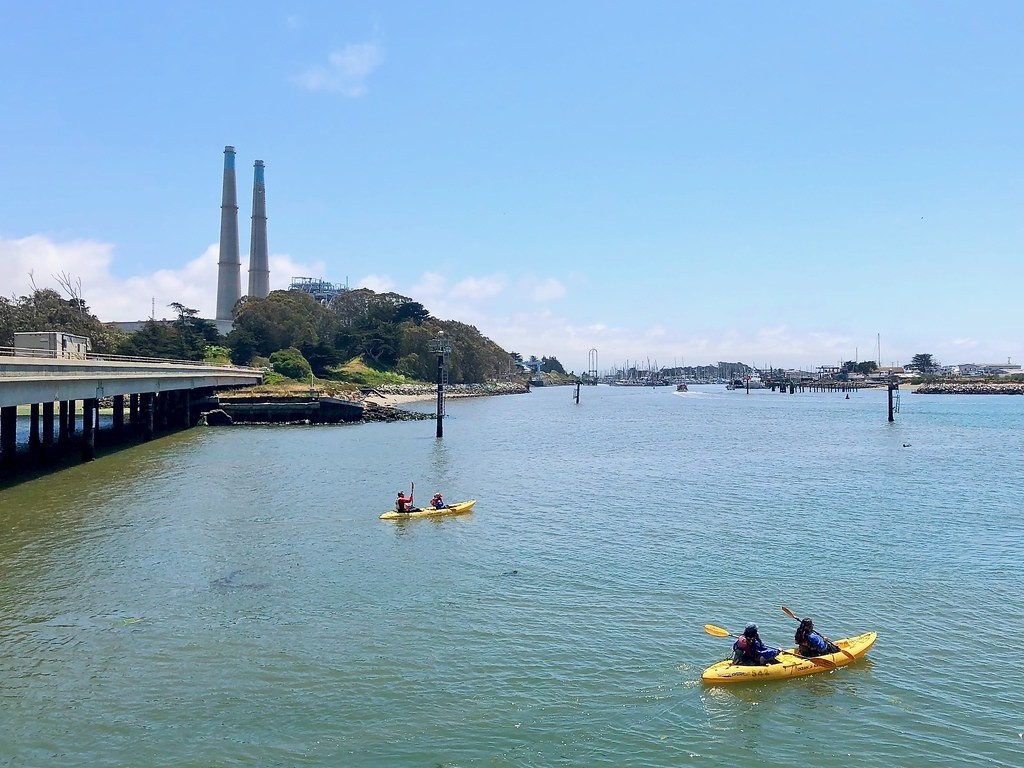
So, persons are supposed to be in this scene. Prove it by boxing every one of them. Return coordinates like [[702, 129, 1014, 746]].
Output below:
[[430, 493, 458, 510], [395, 490, 424, 513], [794, 617, 840, 657], [732, 621, 783, 666]]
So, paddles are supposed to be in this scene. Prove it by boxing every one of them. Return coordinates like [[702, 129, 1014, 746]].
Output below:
[[411, 482, 414, 508], [449, 506, 456, 511], [704, 624, 837, 671], [781, 605, 856, 662]]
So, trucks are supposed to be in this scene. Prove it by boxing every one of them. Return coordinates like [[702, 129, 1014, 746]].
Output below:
[[12, 330, 92, 360]]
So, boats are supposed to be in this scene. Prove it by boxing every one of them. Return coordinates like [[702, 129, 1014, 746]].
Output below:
[[376, 497, 479, 524], [677, 384, 687, 392], [700, 630, 877, 682]]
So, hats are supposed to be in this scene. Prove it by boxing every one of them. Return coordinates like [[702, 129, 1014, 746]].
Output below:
[[802, 617, 812, 622], [745, 623, 759, 629], [435, 493, 443, 497], [398, 491, 405, 495]]
